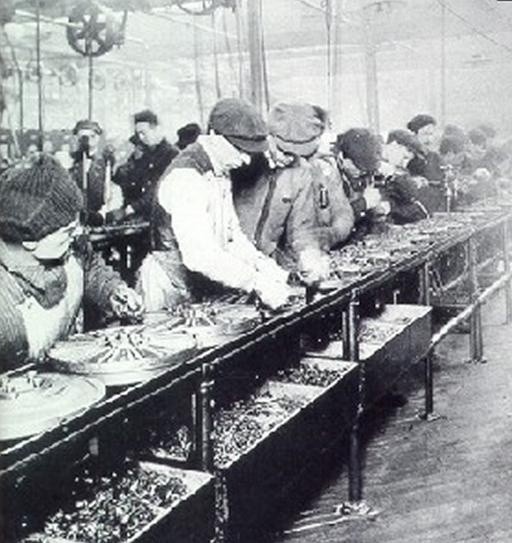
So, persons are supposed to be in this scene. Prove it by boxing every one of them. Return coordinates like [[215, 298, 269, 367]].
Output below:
[[0, 150, 146, 377], [69, 98, 512, 312]]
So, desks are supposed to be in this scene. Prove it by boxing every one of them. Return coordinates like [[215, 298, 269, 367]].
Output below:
[[0, 200, 511, 543]]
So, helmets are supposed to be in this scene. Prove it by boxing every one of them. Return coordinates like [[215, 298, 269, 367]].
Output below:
[[134, 110, 158, 125]]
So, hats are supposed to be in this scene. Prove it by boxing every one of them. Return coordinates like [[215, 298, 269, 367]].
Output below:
[[1, 151, 85, 241], [210, 96, 269, 154], [269, 97, 325, 156], [336, 127, 383, 173], [73, 119, 101, 135], [387, 113, 497, 162]]
